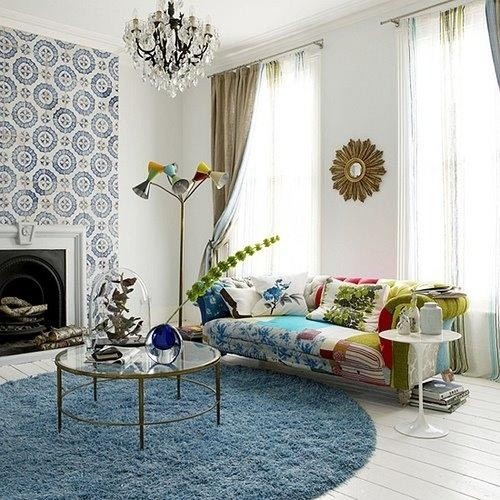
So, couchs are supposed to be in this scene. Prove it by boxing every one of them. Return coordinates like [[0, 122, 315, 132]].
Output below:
[[196, 274, 470, 407]]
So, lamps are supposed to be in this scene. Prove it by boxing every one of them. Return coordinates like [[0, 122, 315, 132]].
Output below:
[[132, 160, 229, 400], [120, 1, 223, 97]]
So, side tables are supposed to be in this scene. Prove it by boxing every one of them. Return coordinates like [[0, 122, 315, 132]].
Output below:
[[379, 328, 463, 438]]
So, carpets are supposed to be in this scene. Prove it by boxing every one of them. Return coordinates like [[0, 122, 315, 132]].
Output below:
[[0, 364, 377, 500]]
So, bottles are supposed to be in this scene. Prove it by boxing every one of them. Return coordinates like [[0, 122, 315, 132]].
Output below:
[[407, 304, 420, 333], [419, 301, 446, 335]]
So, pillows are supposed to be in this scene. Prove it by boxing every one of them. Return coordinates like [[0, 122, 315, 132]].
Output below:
[[307, 276, 390, 332], [219, 286, 262, 319], [249, 274, 311, 317]]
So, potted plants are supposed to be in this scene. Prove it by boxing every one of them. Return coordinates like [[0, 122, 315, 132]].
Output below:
[[145, 236, 279, 365]]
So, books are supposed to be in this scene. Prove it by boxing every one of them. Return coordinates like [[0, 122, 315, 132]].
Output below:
[[92, 346, 122, 362], [412, 282, 468, 300], [410, 379, 472, 413]]
